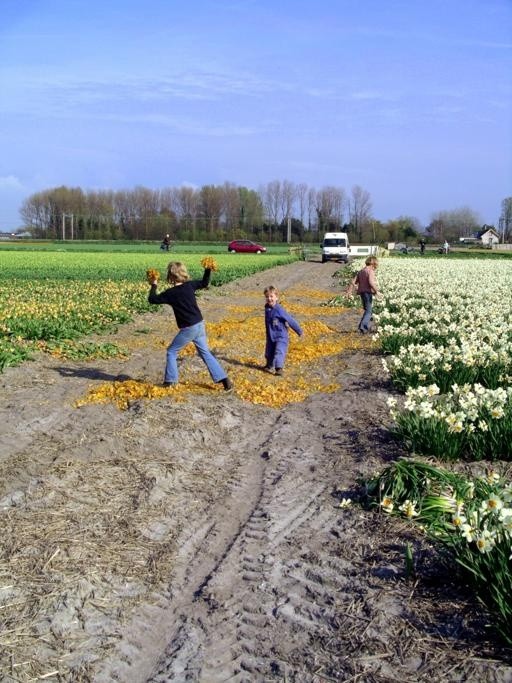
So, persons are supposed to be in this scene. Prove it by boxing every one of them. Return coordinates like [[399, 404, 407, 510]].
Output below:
[[147, 257, 236, 392], [442, 240, 449, 255], [160, 234, 171, 253], [262, 285, 306, 376], [354, 256, 381, 334], [418, 238, 428, 253]]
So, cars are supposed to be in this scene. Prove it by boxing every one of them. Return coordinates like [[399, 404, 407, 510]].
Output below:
[[228, 239, 267, 254]]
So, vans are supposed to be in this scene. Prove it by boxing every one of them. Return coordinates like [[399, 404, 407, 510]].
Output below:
[[320, 232, 351, 263]]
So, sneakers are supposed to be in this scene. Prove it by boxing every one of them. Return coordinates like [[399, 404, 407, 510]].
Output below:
[[222, 378, 233, 390]]
